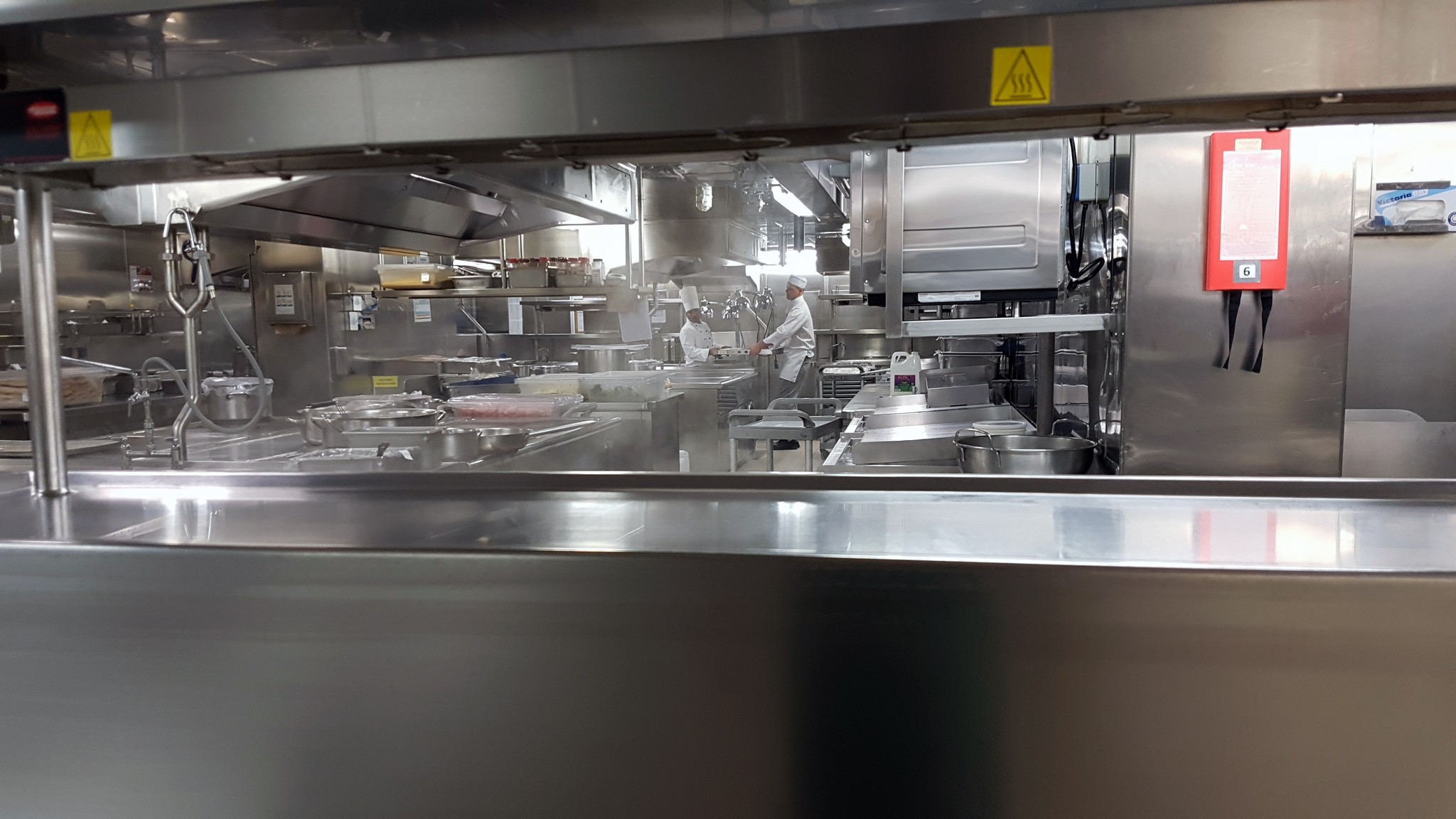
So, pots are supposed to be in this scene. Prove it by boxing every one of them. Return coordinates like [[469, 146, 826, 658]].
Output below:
[[332, 393, 444, 409], [530, 366, 565, 375], [509, 364, 530, 378], [206, 376, 274, 421], [285, 406, 343, 445], [628, 360, 662, 370], [479, 420, 597, 451], [311, 408, 447, 448], [570, 348, 636, 374], [442, 428, 482, 461], [952, 419, 1098, 475]]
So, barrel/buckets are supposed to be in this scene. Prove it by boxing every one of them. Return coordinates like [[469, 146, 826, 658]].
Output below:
[[972, 420, 1027, 436]]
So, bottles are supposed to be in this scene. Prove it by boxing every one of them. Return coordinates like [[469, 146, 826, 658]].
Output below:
[[890, 351, 921, 397], [557, 257, 577, 270], [508, 258, 539, 269], [539, 257, 557, 267], [578, 257, 592, 274], [592, 259, 606, 286]]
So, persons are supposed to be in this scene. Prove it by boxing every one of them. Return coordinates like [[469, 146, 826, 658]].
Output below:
[[749, 274, 815, 450], [679, 286, 731, 367]]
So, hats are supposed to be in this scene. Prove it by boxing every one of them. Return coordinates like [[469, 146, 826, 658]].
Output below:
[[789, 274, 808, 289], [679, 285, 700, 313]]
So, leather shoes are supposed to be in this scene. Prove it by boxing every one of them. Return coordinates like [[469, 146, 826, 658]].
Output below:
[[773, 438, 800, 450]]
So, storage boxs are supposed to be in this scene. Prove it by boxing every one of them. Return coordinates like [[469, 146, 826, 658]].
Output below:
[[373, 261, 592, 289], [443, 369, 673, 421]]
